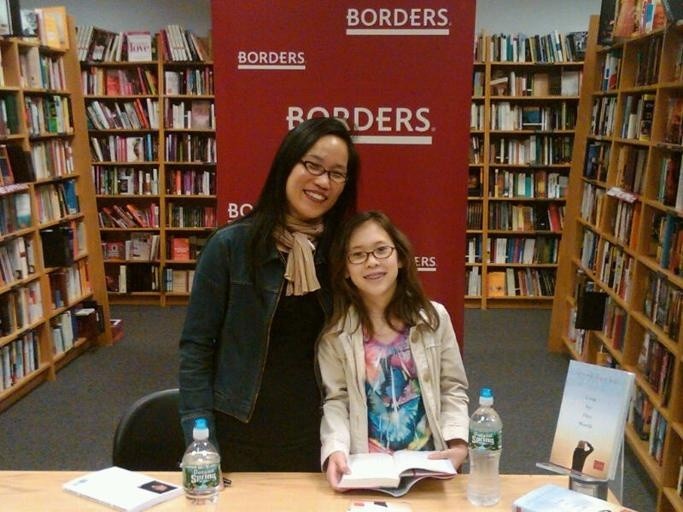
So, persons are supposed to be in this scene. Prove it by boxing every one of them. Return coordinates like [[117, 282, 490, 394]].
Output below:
[[175, 117, 361, 473], [312, 210, 472, 494]]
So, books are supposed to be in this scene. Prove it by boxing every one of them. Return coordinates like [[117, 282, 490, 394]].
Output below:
[[548, 358, 637, 481], [347, 498, 413, 512], [566, 1, 683, 498], [510, 482, 635, 512], [1, 2, 105, 395], [59, 466, 184, 510], [107, 24, 216, 293], [335, 447, 458, 497], [463, 32, 567, 297]]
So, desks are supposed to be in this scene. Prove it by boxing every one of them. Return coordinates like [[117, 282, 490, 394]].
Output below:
[[1, 468, 622, 512]]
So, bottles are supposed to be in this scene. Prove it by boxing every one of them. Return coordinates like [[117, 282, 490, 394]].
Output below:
[[183, 419, 225, 512], [467, 388, 502, 506]]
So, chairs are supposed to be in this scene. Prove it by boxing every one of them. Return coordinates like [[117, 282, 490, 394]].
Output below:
[[111, 389, 184, 471]]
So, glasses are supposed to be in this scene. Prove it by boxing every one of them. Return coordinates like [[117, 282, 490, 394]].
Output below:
[[300, 159, 348, 184], [346, 246, 396, 264]]
